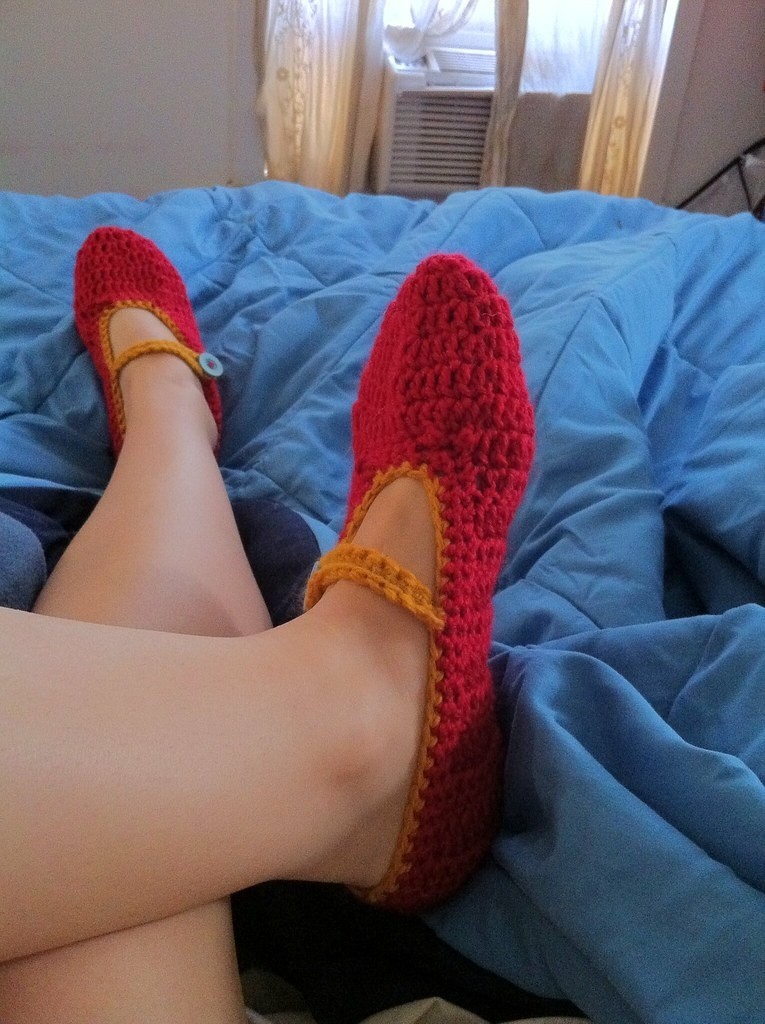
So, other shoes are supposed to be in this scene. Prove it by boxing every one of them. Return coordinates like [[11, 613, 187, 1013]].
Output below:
[[73, 228, 224, 459], [300, 256, 537, 904]]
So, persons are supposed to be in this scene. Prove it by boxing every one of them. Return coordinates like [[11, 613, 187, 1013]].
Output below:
[[0, 222, 538, 1024]]
